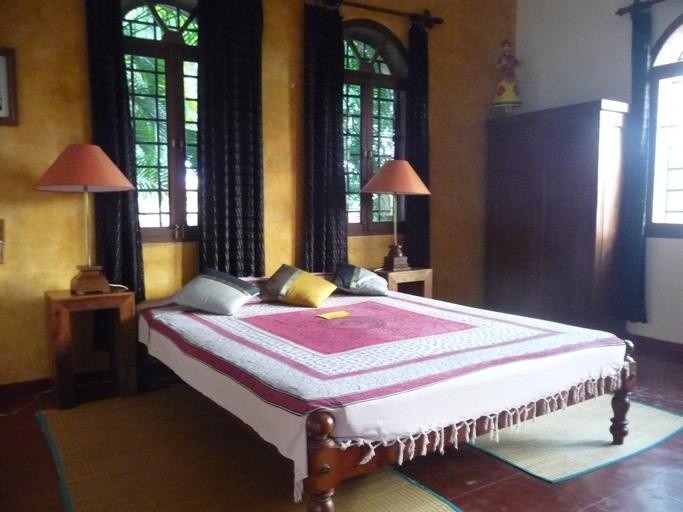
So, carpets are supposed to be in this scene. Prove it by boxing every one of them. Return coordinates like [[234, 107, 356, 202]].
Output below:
[[459, 392, 683, 486], [29, 381, 464, 511]]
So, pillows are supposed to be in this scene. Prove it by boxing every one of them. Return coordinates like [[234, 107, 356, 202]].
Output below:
[[167, 260, 389, 316]]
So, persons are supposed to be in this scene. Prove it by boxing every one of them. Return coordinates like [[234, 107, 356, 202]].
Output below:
[[491, 40, 523, 108]]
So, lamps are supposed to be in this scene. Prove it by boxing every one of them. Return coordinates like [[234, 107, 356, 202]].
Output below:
[[359, 158, 433, 271], [33, 143, 140, 295]]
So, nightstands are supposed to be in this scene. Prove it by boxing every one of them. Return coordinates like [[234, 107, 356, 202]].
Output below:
[[43, 288, 142, 411], [369, 264, 432, 298]]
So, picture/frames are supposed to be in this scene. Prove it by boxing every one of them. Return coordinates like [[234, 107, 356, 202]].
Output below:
[[0, 46, 19, 127]]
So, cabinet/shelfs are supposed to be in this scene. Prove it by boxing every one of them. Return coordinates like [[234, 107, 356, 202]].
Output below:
[[481, 97, 630, 345]]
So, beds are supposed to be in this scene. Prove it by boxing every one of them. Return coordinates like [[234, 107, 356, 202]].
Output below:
[[134, 269, 640, 512]]
